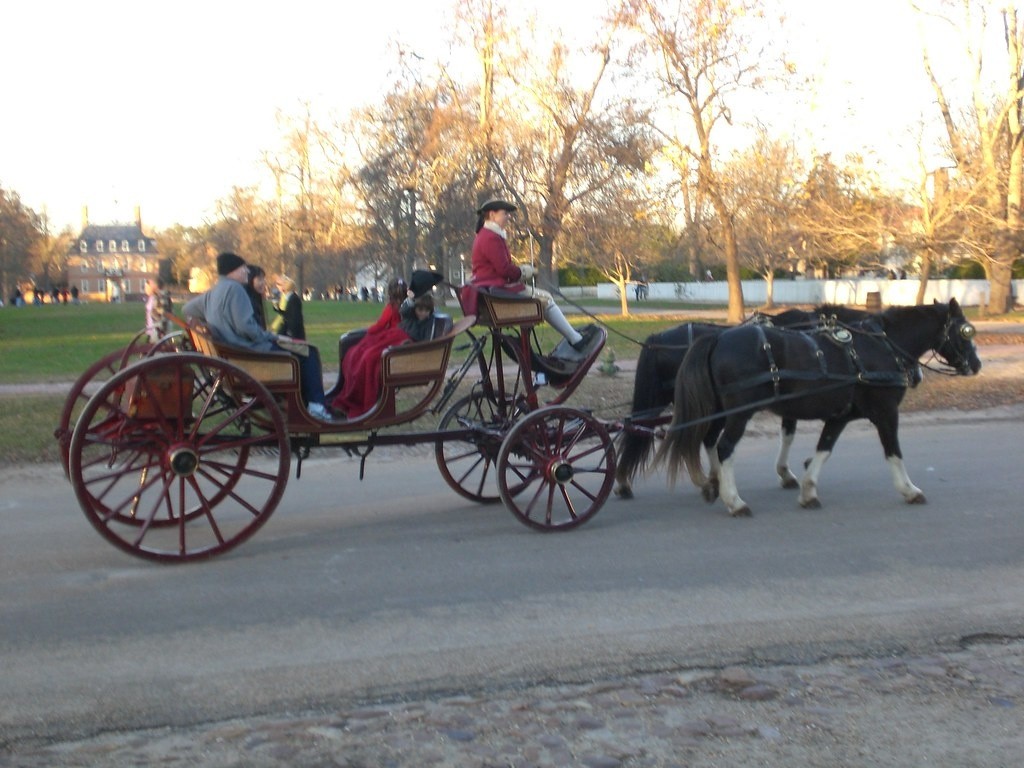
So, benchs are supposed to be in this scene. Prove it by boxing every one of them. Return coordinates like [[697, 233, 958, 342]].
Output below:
[[158, 306, 302, 422], [333, 312, 481, 425]]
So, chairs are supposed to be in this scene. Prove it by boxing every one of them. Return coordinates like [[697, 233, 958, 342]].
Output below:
[[447, 280, 543, 327]]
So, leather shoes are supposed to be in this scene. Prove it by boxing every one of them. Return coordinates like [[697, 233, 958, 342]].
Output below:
[[567, 322, 596, 351]]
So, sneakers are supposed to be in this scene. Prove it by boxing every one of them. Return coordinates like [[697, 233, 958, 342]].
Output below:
[[307, 402, 332, 420]]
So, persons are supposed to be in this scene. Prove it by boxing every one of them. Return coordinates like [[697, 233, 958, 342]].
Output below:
[[143, 279, 173, 358], [472, 199, 598, 350], [180, 252, 332, 420], [0, 285, 79, 306], [332, 277, 437, 419]]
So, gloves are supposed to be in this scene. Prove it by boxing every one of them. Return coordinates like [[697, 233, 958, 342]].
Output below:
[[521, 264, 533, 280]]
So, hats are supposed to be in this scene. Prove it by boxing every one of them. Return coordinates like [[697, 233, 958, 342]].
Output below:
[[476, 201, 517, 215], [217, 253, 245, 276], [409, 270, 444, 299]]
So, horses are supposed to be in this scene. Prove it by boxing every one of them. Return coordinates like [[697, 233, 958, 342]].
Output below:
[[647, 296, 982, 518], [613, 304, 923, 499]]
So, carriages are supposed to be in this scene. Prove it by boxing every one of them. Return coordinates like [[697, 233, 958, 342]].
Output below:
[[52, 268, 983, 567]]
